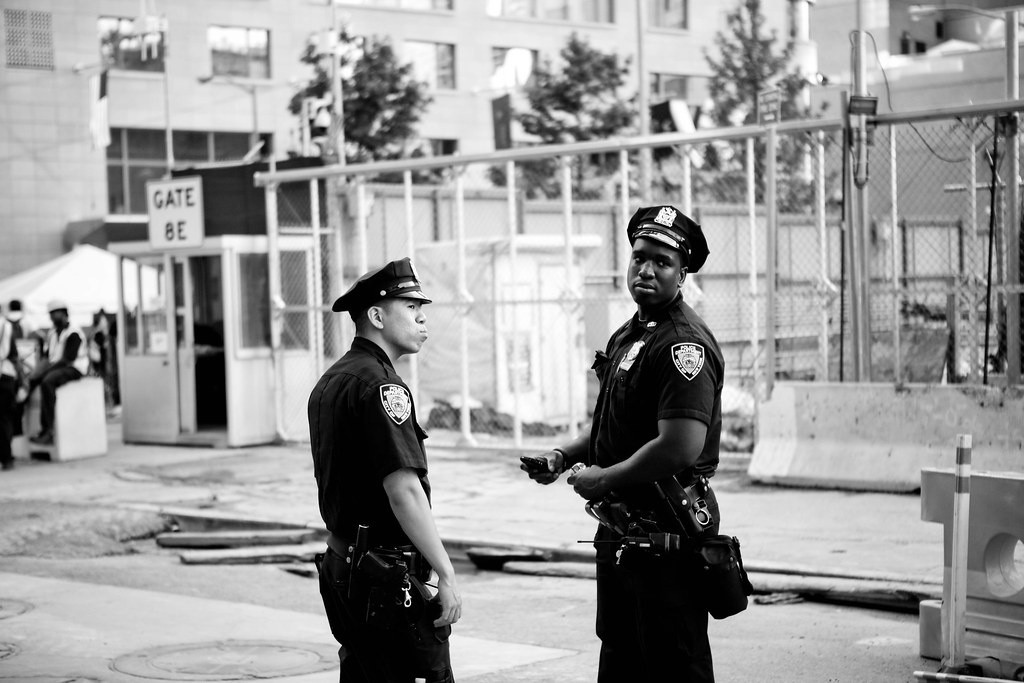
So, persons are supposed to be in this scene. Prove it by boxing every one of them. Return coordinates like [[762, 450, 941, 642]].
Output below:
[[308, 256, 462, 683], [0, 299, 131, 471], [520, 206, 725, 682]]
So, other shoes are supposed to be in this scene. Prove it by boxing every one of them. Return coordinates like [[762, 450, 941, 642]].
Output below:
[[29, 430, 51, 444]]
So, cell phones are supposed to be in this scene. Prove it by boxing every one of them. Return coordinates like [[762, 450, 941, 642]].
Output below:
[[520, 456, 550, 473]]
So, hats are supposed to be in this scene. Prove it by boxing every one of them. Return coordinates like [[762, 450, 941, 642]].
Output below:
[[48, 300, 69, 313], [332, 256, 431, 317], [626, 205, 710, 273]]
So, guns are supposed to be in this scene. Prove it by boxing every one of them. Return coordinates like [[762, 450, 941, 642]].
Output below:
[[356, 553, 409, 596]]
